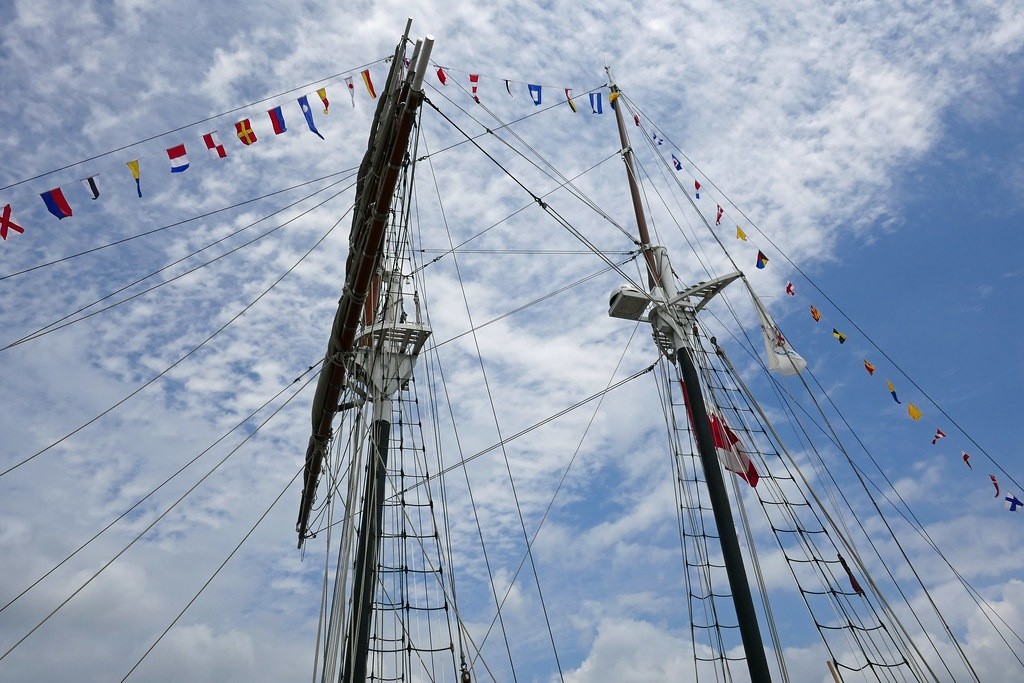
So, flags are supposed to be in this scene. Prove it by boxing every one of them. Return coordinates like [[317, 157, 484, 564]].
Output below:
[[0, 66, 604, 240], [609, 91, 1024, 512], [744, 276, 807, 375], [680, 380, 758, 488]]
